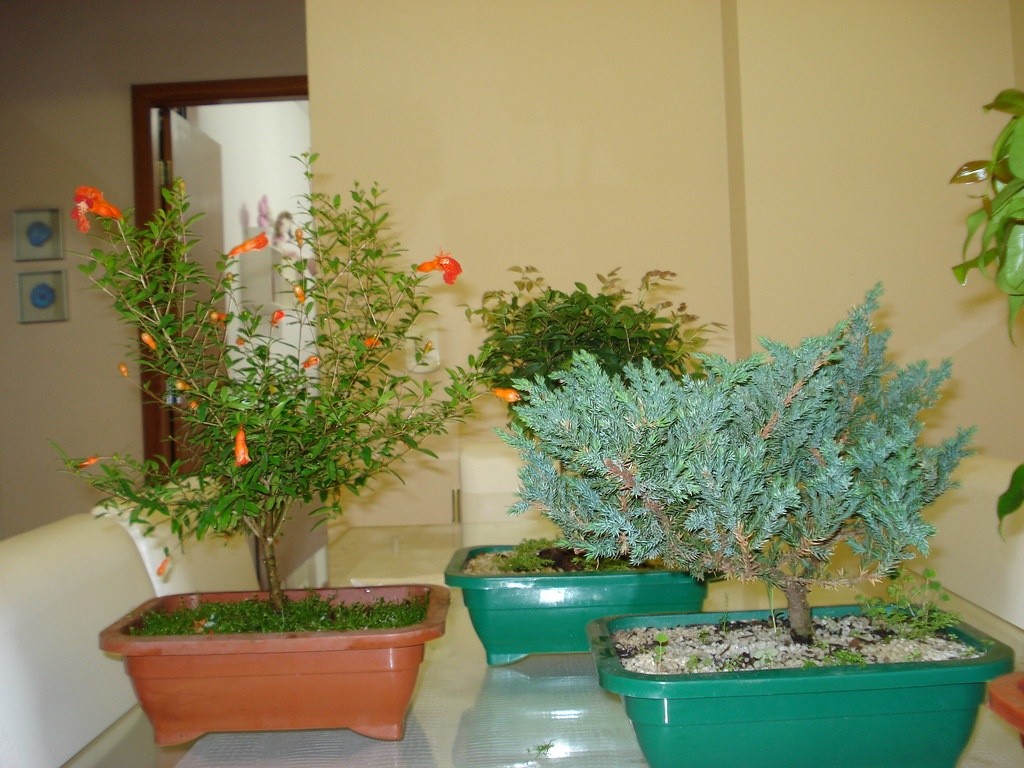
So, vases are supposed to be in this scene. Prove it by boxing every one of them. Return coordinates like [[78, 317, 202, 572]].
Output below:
[[98, 580, 454, 748]]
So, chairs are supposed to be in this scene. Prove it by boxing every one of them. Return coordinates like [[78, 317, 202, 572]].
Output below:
[[2, 476, 268, 767]]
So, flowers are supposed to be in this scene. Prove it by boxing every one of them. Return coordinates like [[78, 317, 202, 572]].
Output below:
[[63, 156, 481, 619]]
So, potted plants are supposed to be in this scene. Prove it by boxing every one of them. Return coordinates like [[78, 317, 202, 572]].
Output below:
[[491, 277, 1015, 768], [442, 264, 729, 672]]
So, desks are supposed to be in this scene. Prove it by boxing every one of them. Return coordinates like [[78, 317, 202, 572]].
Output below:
[[63, 509, 1022, 767]]
